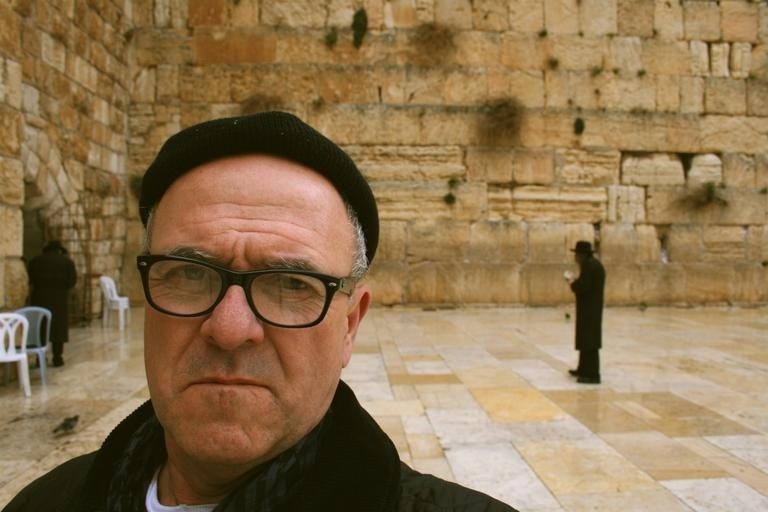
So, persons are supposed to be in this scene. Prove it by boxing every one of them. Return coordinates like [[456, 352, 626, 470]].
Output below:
[[562, 239, 612, 384], [0, 111, 520, 512], [25, 237, 76, 367]]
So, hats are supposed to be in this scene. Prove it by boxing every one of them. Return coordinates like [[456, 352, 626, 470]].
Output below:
[[138, 112, 379, 266], [42, 240, 68, 254], [570, 242, 595, 253]]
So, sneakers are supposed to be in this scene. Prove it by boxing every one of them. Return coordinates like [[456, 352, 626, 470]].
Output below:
[[569, 370, 600, 383], [37, 356, 63, 368]]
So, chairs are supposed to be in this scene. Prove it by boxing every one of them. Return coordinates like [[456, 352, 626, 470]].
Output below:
[[99, 274, 130, 330], [0, 306, 54, 398]]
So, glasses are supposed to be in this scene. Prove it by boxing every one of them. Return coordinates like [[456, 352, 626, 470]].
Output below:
[[136, 253, 357, 329]]
